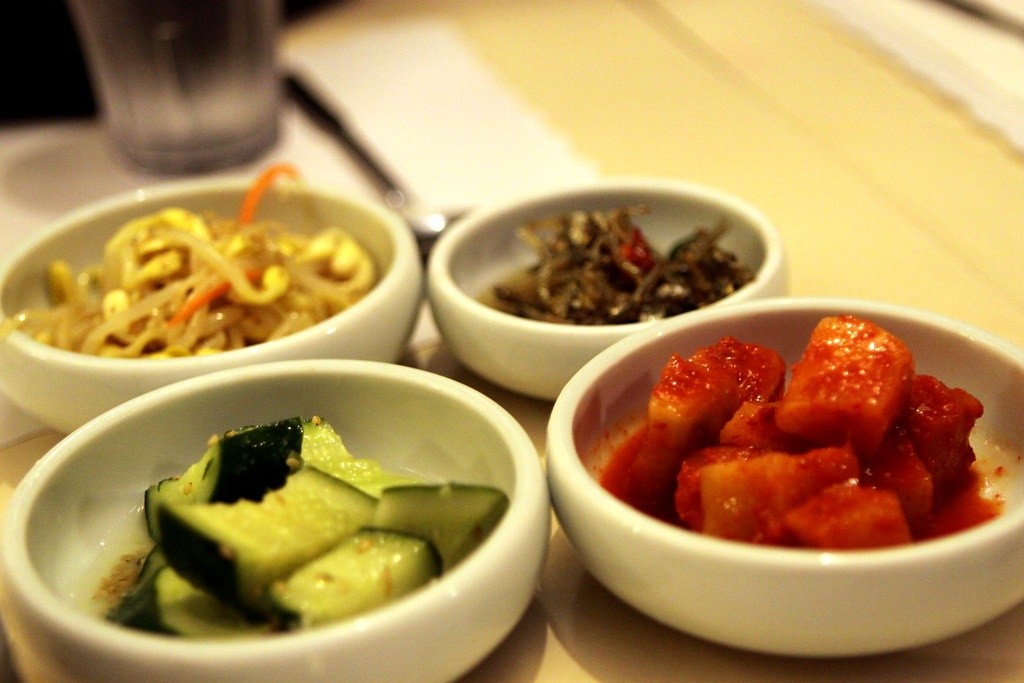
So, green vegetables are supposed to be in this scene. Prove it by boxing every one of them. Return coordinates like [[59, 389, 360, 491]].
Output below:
[[481, 207, 752, 327]]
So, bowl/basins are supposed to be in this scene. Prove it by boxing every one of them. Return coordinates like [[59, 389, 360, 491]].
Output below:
[[0, 174, 423, 436], [5, 359, 550, 683], [544, 296, 1024, 656], [425, 179, 787, 398]]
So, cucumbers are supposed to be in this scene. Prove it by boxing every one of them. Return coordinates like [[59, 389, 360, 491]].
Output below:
[[105, 411, 507, 641]]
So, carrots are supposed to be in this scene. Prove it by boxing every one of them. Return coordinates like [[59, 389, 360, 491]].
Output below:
[[236, 160, 299, 229]]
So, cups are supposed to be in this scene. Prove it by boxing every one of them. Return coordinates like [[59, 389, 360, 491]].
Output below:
[[71, 0, 281, 174]]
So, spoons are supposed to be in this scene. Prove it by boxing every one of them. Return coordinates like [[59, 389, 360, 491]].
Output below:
[[283, 70, 473, 254]]
